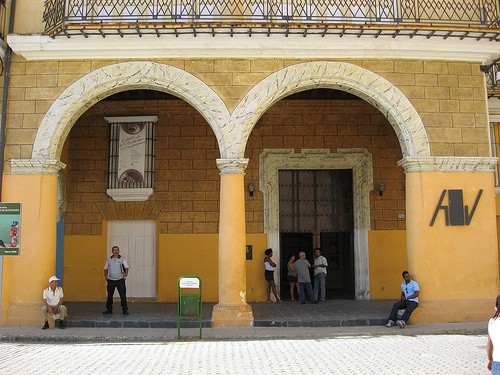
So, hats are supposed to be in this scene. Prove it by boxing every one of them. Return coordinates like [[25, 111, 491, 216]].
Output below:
[[48, 276, 60, 283]]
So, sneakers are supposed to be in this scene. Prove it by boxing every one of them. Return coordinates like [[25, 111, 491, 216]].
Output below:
[[396, 320, 405, 329], [386, 320, 396, 327]]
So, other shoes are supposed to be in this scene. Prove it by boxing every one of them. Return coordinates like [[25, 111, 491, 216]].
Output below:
[[41, 320, 49, 330], [312, 301, 318, 304], [123, 311, 129, 315], [102, 311, 113, 315], [291, 299, 295, 304], [301, 301, 305, 304], [277, 300, 285, 304], [267, 301, 275, 304], [59, 320, 65, 329], [319, 300, 325, 304]]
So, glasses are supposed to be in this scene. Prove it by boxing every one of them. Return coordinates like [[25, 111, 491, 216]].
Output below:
[[406, 288, 408, 293]]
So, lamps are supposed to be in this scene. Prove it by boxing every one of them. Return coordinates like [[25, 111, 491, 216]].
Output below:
[[248, 183, 255, 197], [377, 181, 385, 196]]
